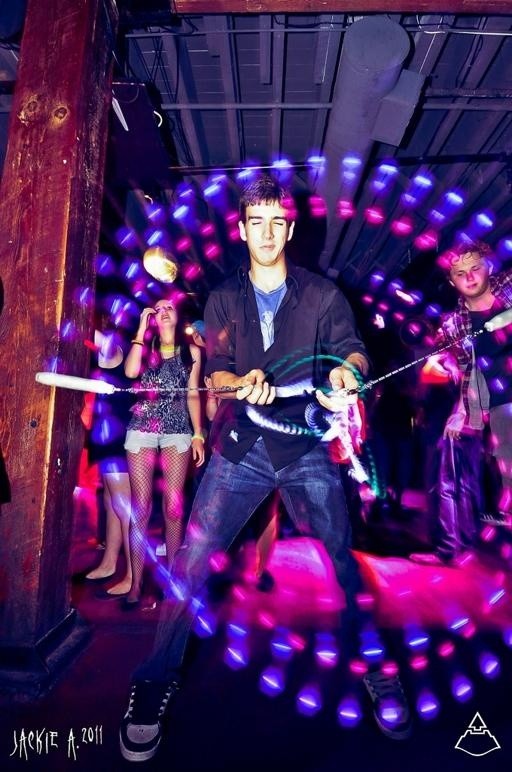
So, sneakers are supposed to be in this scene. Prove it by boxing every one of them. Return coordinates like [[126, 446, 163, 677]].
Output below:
[[356, 657, 416, 741], [409, 551, 442, 566], [119, 666, 182, 763]]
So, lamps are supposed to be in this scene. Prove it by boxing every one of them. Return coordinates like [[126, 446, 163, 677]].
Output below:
[[110, 36, 155, 132]]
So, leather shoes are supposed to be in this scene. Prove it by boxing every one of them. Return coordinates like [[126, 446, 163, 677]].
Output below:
[[120, 598, 139, 610], [71, 567, 113, 585], [92, 587, 129, 600]]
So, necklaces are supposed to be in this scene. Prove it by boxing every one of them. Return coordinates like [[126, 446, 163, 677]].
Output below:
[[159, 338, 174, 346]]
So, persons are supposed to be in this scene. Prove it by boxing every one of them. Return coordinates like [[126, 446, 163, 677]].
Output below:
[[113, 296, 207, 622], [423, 236, 511, 568], [408, 382, 488, 566], [199, 355, 279, 597], [112, 172, 417, 761], [71, 310, 146, 602]]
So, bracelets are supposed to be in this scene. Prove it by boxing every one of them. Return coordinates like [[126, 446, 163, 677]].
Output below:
[[189, 433, 205, 443], [129, 339, 145, 346]]
